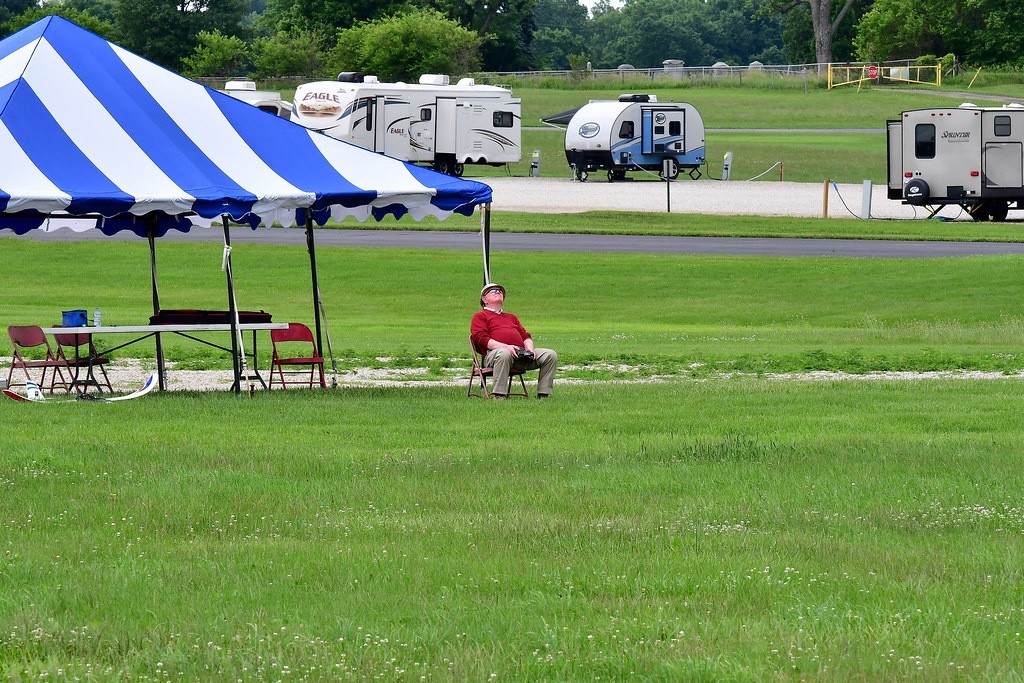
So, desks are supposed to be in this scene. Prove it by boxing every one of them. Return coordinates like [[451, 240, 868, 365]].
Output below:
[[43, 322, 289, 395]]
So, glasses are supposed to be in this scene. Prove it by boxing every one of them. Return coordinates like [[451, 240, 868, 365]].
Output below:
[[484, 288, 503, 296]]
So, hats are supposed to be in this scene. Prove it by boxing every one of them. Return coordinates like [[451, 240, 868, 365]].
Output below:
[[480, 283, 506, 307]]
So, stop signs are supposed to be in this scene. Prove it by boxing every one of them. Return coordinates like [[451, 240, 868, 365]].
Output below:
[[868, 66, 878, 78]]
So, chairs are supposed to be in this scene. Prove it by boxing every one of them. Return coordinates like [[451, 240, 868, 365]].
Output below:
[[467, 335, 528, 399], [8, 325, 70, 396], [51, 325, 113, 395], [270, 322, 326, 390]]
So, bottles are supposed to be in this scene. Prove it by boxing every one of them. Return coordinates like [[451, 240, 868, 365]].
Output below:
[[93, 308, 101, 327]]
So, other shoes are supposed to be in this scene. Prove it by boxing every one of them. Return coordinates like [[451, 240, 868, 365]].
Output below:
[[493, 396, 506, 400]]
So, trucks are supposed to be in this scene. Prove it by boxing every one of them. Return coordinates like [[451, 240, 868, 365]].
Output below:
[[883, 101, 1023, 219], [539, 91, 707, 182], [216, 70, 523, 176]]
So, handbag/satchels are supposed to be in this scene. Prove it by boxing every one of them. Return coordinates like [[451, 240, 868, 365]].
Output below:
[[62, 310, 87, 327]]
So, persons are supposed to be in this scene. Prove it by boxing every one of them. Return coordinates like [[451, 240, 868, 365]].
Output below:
[[470, 283, 557, 400]]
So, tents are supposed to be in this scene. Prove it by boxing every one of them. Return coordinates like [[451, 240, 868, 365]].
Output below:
[[0, 16, 493, 397]]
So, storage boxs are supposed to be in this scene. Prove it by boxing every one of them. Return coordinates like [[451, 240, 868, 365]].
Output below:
[[62, 310, 88, 327], [0, 379, 7, 388]]
